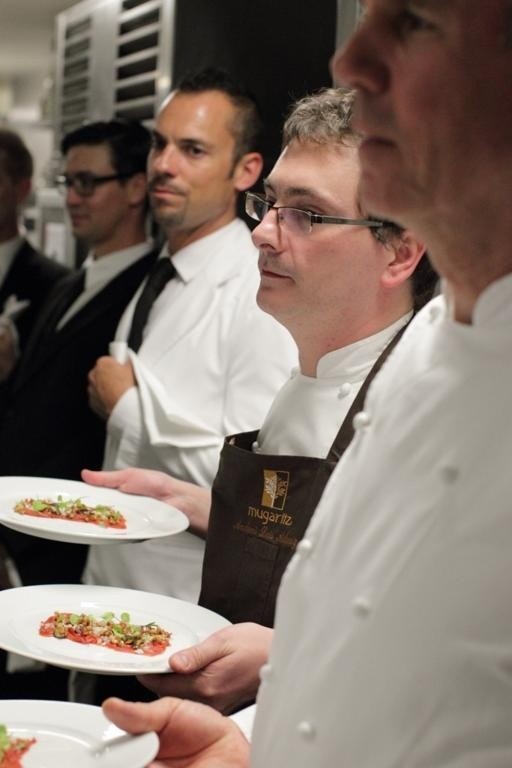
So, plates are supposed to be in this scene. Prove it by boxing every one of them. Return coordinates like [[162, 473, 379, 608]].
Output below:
[[1, 475, 190, 547], [0, 583, 237, 676], [1, 699, 160, 766]]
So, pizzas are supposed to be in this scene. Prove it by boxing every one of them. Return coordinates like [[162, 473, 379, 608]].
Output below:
[[15, 496, 127, 531], [0, 722, 38, 767], [39, 608, 171, 659]]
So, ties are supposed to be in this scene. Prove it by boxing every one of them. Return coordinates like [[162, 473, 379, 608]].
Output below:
[[127, 258, 175, 356]]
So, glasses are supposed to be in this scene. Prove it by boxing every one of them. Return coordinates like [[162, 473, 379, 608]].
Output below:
[[57, 172, 124, 199], [243, 193, 384, 236]]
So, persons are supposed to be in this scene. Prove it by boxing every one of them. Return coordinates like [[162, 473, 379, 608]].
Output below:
[[97, 0, 512, 768], [1, 66, 446, 720]]
